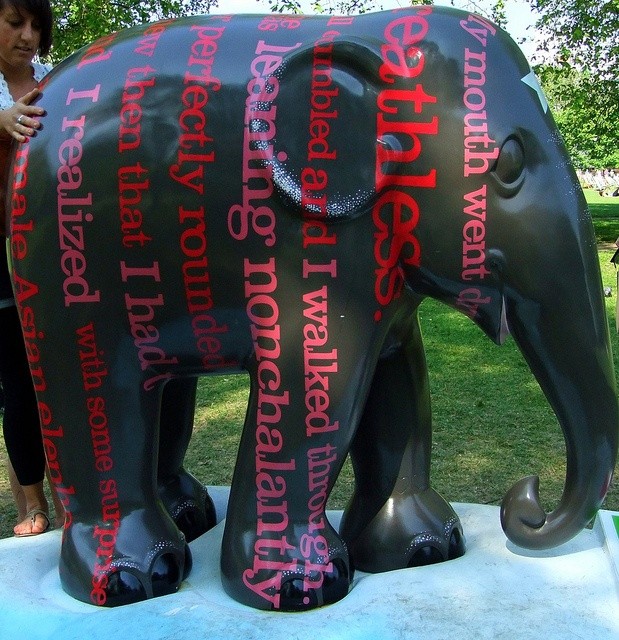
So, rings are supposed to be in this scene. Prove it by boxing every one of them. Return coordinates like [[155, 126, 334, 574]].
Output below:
[[17, 115, 25, 124]]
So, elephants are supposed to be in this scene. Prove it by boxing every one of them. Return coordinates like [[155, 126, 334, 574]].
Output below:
[[0, 2, 619, 615]]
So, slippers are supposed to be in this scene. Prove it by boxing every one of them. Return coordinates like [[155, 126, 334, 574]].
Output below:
[[14, 508, 50, 538]]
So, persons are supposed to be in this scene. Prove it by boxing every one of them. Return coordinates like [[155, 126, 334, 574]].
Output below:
[[0, 0, 66, 535]]
